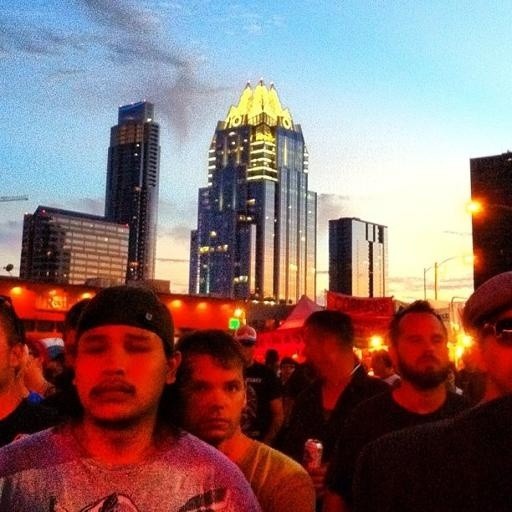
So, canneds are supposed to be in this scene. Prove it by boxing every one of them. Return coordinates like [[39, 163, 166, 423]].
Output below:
[[303, 438, 324, 471]]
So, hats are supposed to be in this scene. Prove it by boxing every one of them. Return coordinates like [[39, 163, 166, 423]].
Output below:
[[78, 286, 173, 359], [462, 271, 512, 337]]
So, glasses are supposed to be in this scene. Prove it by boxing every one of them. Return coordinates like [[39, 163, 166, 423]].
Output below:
[[494, 317, 512, 339]]
